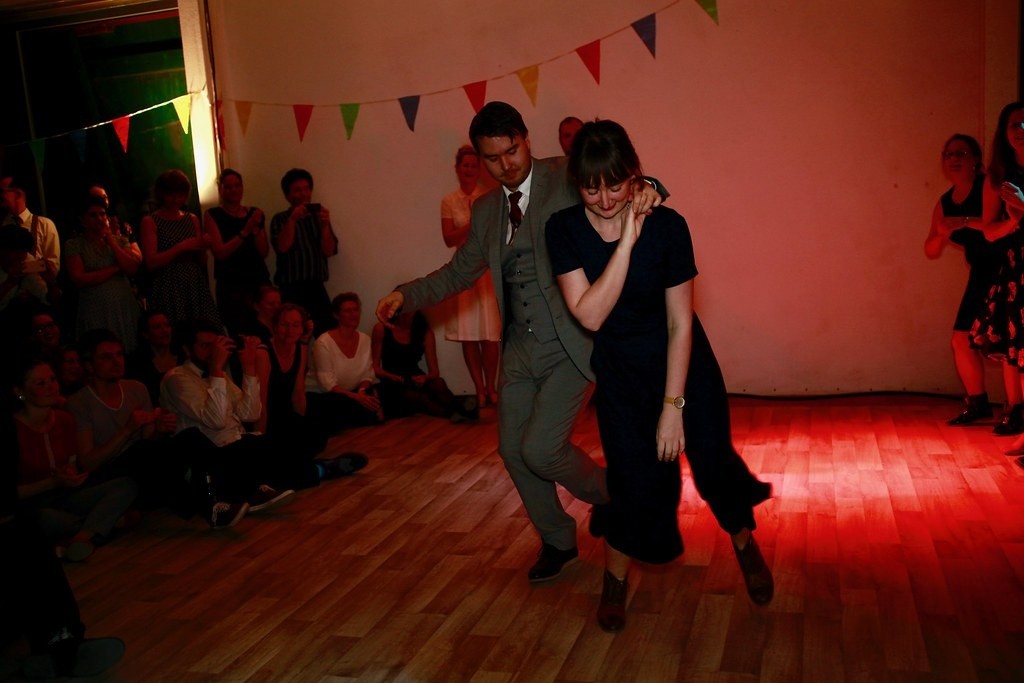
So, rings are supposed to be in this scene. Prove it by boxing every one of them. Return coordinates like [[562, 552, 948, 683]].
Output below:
[[627, 205, 630, 209]]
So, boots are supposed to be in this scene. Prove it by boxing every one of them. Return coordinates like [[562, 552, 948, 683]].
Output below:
[[731, 532, 773, 607], [596, 568, 628, 633]]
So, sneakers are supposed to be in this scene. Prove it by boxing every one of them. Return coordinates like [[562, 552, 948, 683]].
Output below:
[[249, 485, 296, 517], [210, 497, 252, 529]]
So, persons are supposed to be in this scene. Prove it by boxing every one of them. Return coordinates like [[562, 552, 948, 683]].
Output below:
[[557, 117, 586, 155], [441, 143, 500, 408], [0, 160, 498, 683], [372, 99, 671, 580], [967, 99, 1024, 463], [544, 119, 773, 631], [924, 133, 1024, 435]]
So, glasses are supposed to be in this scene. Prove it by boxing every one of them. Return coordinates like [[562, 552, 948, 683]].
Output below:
[[940, 149, 970, 161], [1006, 121, 1024, 131]]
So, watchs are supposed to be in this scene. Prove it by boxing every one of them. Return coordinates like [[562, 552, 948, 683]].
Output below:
[[643, 179, 656, 188], [664, 396, 686, 409]]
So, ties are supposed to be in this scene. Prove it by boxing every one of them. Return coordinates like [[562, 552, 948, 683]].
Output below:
[[507, 190, 524, 245]]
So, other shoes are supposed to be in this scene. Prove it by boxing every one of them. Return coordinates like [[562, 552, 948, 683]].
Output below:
[[528, 543, 578, 582], [948, 391, 993, 424], [993, 400, 1023, 435], [588, 502, 612, 537], [55, 538, 94, 563], [18, 635, 125, 681], [318, 451, 367, 478], [1003, 434, 1024, 454]]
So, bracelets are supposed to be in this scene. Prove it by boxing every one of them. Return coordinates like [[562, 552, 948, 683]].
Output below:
[[963, 216, 969, 227]]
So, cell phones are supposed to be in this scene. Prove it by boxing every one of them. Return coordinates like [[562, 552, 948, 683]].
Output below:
[[227, 337, 244, 353]]
